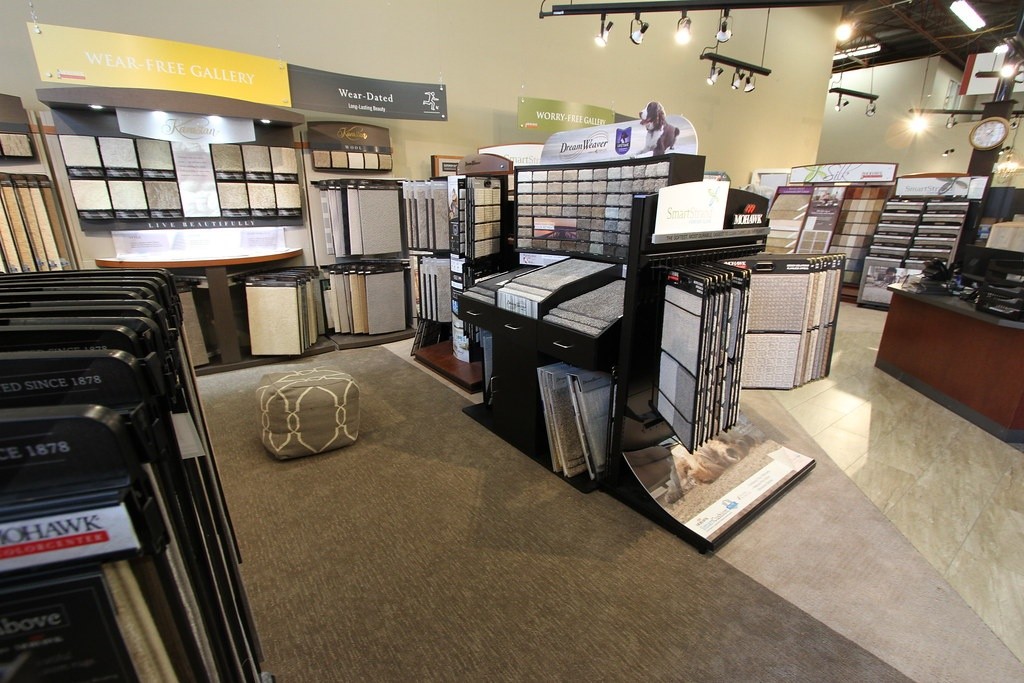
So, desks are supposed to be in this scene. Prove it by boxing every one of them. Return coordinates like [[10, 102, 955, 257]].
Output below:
[[875, 283, 1024, 453]]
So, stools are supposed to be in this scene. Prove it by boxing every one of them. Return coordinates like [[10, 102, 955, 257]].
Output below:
[[254, 367, 359, 462]]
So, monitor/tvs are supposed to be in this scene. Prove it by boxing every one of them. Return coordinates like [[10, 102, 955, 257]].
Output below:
[[960, 244, 1024, 313]]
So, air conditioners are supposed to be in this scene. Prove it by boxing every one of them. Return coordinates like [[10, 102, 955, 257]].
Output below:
[[707, 61, 722, 85]]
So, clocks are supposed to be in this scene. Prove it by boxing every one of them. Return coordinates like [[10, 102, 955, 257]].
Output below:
[[970, 117, 1009, 149]]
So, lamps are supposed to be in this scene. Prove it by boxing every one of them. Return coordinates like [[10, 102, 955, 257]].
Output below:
[[834, 93, 848, 112], [947, 113, 955, 130], [866, 99, 876, 116], [717, 8, 731, 43], [595, 15, 613, 48], [730, 69, 745, 90], [943, 149, 955, 156], [628, 12, 648, 44], [676, 11, 692, 45], [746, 72, 756, 92]]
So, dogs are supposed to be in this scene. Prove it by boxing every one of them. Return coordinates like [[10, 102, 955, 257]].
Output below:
[[634, 101, 680, 157]]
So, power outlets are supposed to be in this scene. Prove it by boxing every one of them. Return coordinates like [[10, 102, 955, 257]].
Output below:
[[1007, 145, 1015, 153], [1011, 118, 1019, 129]]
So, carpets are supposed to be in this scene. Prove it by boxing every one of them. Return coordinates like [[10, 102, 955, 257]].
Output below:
[[193, 345, 913, 683]]
